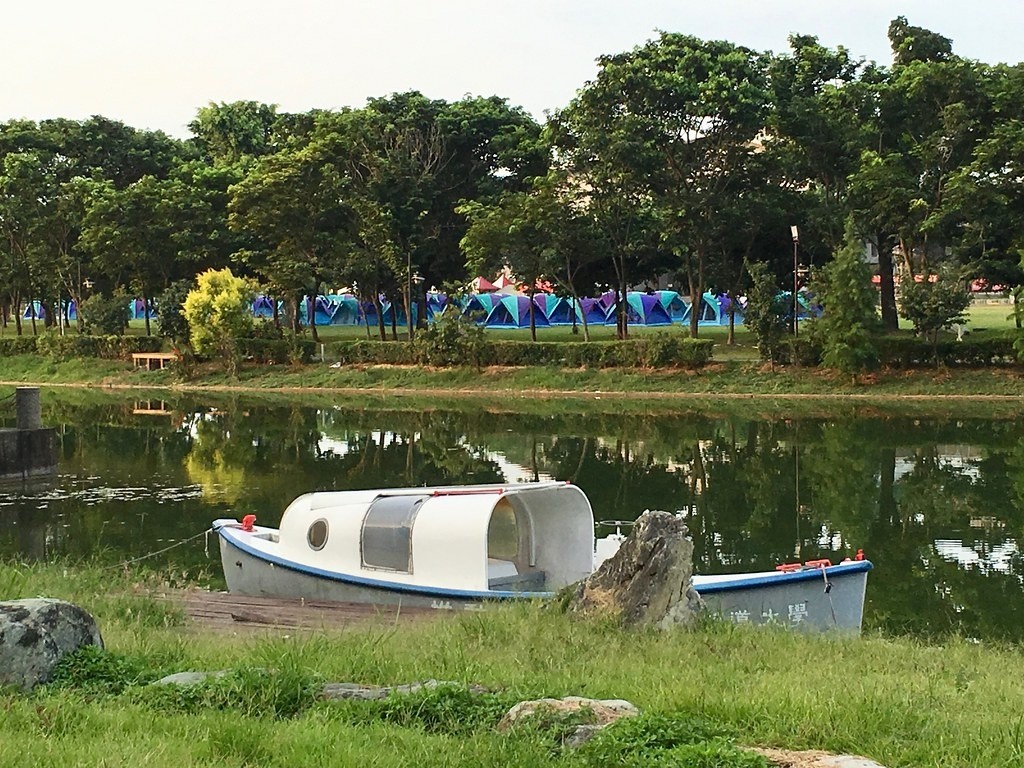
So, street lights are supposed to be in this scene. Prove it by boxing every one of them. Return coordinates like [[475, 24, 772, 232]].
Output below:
[[791, 225, 800, 338]]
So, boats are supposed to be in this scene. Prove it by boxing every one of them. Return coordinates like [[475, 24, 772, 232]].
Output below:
[[213, 484, 870, 645]]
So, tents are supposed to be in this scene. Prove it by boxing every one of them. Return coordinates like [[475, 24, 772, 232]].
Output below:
[[776, 291, 824, 321], [482, 295, 552, 329], [22, 291, 649, 326], [653, 290, 688, 321], [458, 276, 501, 293], [680, 292, 744, 327], [604, 292, 672, 328], [491, 274, 514, 288]]
